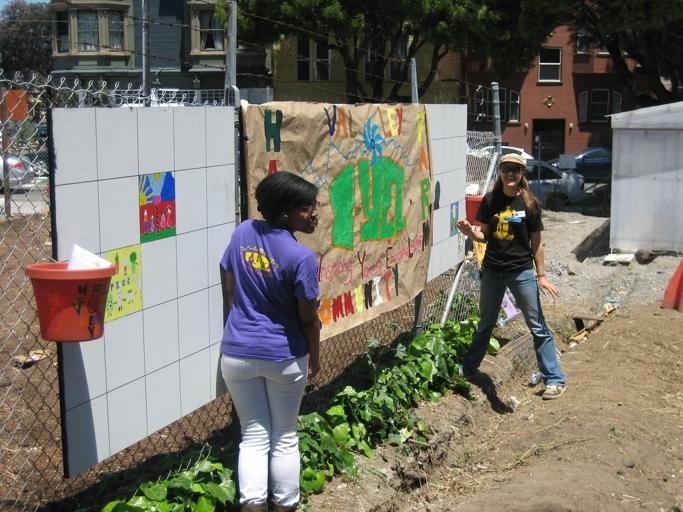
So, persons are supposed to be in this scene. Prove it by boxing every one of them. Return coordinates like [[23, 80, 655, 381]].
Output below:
[[454, 152, 568, 399], [219, 172, 321, 512], [143, 205, 173, 235], [106, 249, 140, 319]]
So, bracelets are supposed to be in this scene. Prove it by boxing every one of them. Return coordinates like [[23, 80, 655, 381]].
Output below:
[[537, 274, 546, 277]]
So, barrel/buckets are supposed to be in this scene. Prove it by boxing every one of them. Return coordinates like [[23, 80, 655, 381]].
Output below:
[[466, 195, 484, 227], [23, 261, 117, 342]]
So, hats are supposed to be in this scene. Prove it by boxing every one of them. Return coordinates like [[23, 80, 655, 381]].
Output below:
[[497, 152, 526, 165]]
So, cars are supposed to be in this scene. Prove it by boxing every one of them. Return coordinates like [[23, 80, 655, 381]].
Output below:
[[0, 126, 49, 185], [466, 145, 611, 207]]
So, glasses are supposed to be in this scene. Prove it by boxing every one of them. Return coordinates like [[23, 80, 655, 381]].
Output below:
[[502, 165, 522, 173]]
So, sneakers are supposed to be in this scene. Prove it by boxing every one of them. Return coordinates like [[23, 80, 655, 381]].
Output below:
[[543, 383, 566, 399], [452, 364, 475, 382]]
